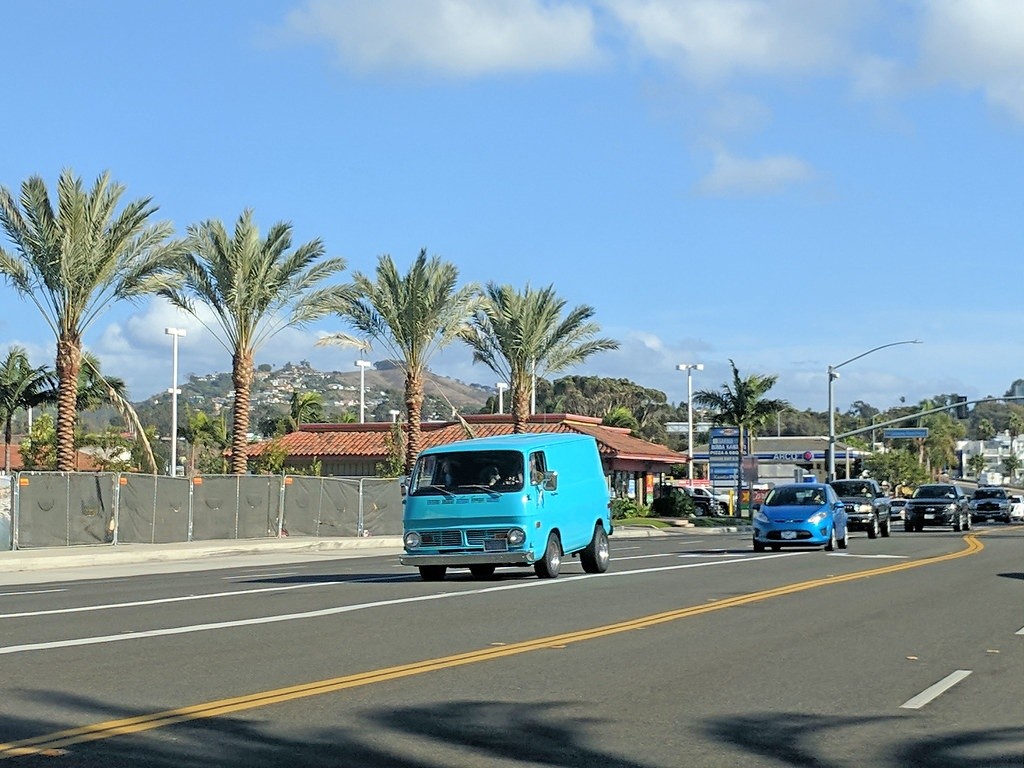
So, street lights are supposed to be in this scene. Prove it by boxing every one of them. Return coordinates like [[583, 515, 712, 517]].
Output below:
[[354, 359, 371, 424], [165, 327, 186, 476], [828, 338, 926, 480], [496, 382, 508, 414], [676, 363, 704, 486]]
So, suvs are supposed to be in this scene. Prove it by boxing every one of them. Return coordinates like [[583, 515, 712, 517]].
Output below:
[[967, 487, 1013, 523], [904, 483, 971, 532], [826, 478, 892, 540]]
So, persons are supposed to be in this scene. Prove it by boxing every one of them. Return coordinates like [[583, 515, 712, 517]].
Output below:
[[806, 491, 825, 505], [940, 488, 956, 498], [439, 455, 462, 491], [786, 492, 800, 504], [854, 483, 870, 497], [480, 452, 507, 485]]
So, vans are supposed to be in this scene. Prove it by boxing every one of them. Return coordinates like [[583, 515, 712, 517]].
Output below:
[[977, 472, 1003, 488], [400, 432, 614, 583]]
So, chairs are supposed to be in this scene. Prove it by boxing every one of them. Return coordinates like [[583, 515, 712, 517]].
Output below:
[[801, 496, 812, 505]]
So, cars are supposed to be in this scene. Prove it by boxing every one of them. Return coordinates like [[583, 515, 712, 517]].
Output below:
[[889, 497, 908, 521], [752, 482, 850, 552], [1008, 495, 1024, 522], [660, 483, 737, 519]]
